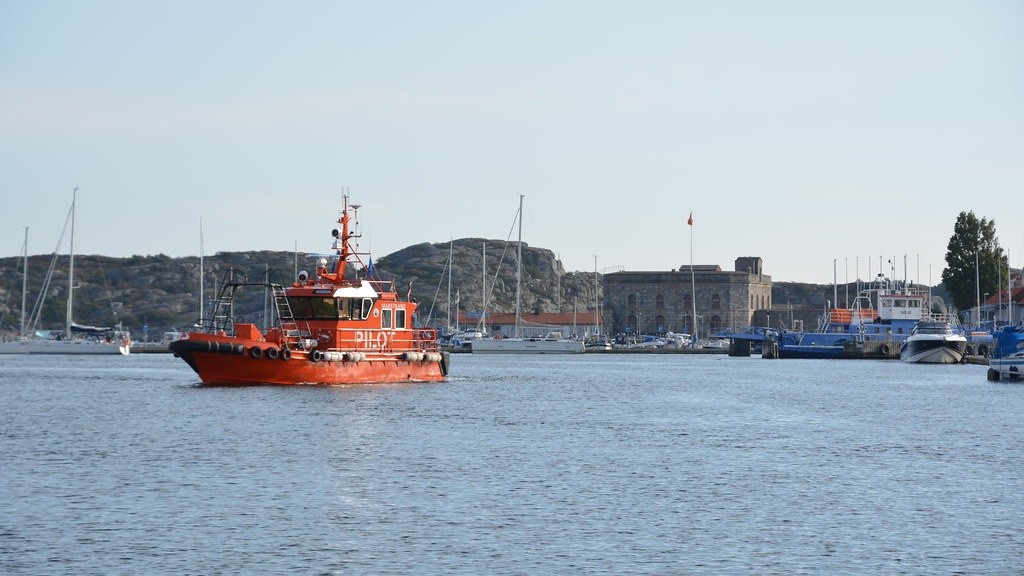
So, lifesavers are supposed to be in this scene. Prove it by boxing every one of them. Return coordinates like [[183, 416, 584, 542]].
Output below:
[[279, 348, 291, 360], [978, 344, 988, 355], [880, 344, 889, 354], [965, 345, 975, 355], [249, 346, 263, 360], [419, 327, 438, 344], [310, 349, 321, 361], [265, 347, 278, 359]]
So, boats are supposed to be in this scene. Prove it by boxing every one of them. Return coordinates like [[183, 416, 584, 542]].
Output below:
[[0, 193, 1024, 383], [167, 186, 450, 384]]
